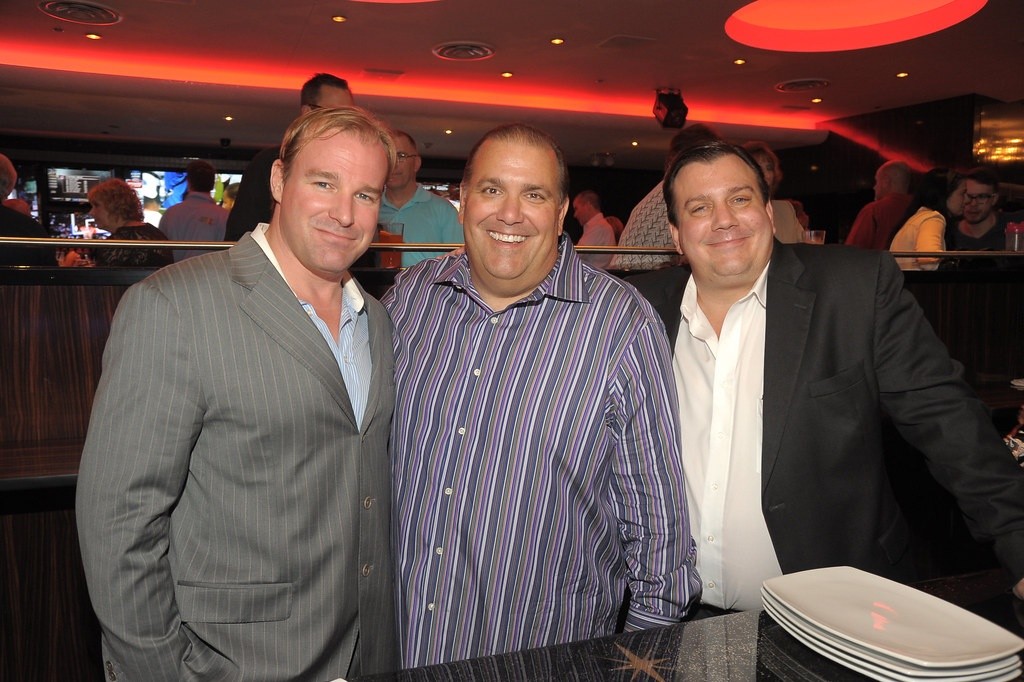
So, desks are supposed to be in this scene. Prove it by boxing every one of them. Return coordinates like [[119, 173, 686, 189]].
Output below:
[[972, 378, 1024, 410], [0, 438, 84, 516], [338, 569, 1023, 682]]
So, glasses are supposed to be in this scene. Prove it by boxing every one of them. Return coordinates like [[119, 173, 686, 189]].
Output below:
[[963, 192, 996, 205], [398, 151, 417, 161]]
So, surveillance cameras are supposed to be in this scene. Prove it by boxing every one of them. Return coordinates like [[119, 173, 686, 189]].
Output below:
[[653, 93, 688, 128], [221, 138, 231, 146]]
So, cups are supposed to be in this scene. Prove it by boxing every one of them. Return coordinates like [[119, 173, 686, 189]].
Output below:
[[56, 252, 64, 266], [799, 231, 825, 244], [80, 227, 94, 239], [33, 201, 38, 210], [377, 223, 404, 269]]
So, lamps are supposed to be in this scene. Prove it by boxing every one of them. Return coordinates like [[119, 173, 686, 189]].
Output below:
[[653, 88, 687, 128], [590, 152, 615, 166]]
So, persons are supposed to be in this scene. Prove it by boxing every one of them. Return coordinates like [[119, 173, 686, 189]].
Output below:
[[608, 124, 725, 270], [378, 123, 703, 669], [844, 161, 924, 250], [0, 154, 59, 284], [944, 168, 1024, 251], [87, 177, 174, 269], [222, 74, 384, 268], [888, 168, 967, 271], [75, 105, 400, 682], [158, 159, 241, 243], [574, 191, 625, 269], [740, 141, 810, 244], [373, 129, 466, 270], [620, 143, 1024, 601]]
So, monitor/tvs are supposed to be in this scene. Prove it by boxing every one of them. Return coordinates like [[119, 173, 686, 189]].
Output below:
[[46, 165, 115, 207]]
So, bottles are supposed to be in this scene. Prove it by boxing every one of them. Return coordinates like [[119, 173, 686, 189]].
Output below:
[[56, 248, 91, 260], [1005, 222, 1024, 251]]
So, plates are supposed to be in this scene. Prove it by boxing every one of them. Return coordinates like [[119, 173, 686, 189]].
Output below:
[[48, 173, 58, 189], [760, 566, 1024, 682]]
